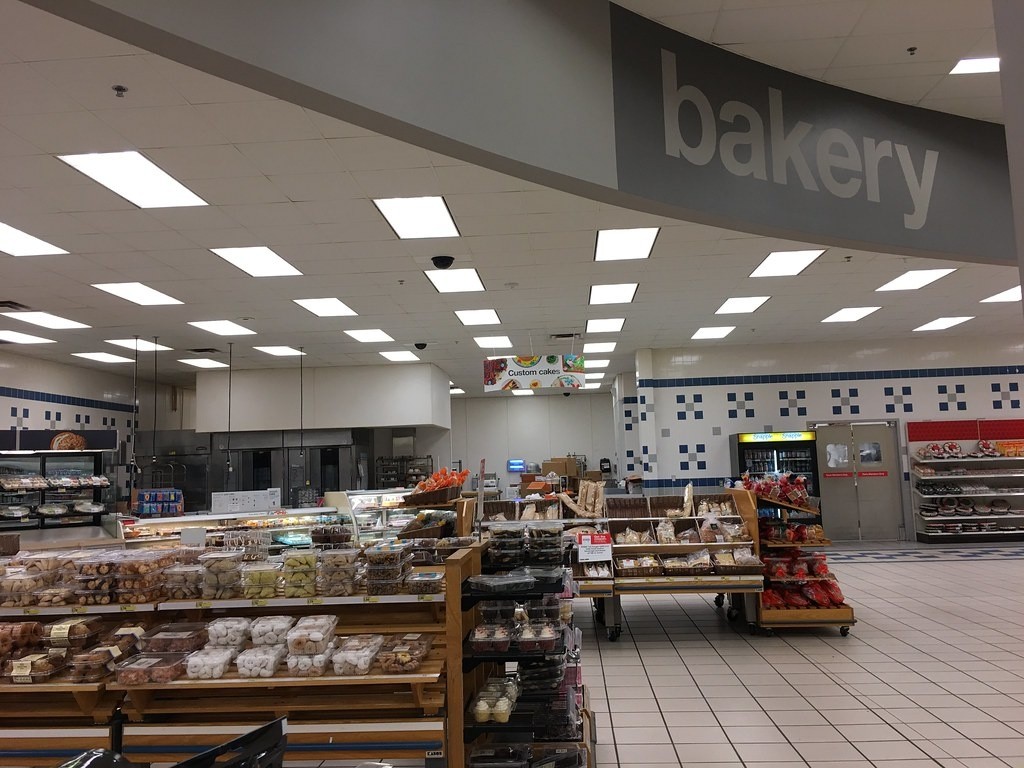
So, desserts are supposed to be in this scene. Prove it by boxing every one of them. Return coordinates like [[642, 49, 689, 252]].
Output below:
[[0, 527, 569, 721]]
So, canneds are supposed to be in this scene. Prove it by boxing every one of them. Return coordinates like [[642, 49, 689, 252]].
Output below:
[[745, 451, 811, 472]]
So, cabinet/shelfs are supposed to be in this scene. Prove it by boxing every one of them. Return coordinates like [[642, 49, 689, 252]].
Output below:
[[725, 488, 859, 637], [375, 455, 435, 488], [608, 492, 763, 640], [115, 507, 337, 551], [0, 538, 490, 768], [905, 418, 1024, 544], [398, 497, 475, 536], [0, 448, 112, 533], [473, 496, 614, 628], [463, 585, 597, 768]]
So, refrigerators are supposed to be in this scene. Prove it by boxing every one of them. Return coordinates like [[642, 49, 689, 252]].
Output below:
[[729, 430, 822, 527]]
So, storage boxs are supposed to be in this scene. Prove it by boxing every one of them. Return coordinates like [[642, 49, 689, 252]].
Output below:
[[467, 521, 591, 768], [520, 459, 603, 498], [0, 539, 443, 689]]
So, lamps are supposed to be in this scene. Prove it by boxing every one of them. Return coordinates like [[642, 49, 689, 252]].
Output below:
[[150, 336, 160, 463], [298, 346, 305, 459], [130, 335, 141, 466], [226, 342, 235, 464]]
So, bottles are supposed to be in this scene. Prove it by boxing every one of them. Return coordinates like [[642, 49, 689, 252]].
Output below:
[[745, 450, 773, 481], [778, 448, 812, 495]]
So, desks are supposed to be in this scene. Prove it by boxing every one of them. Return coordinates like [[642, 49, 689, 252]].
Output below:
[[462, 490, 504, 500]]
